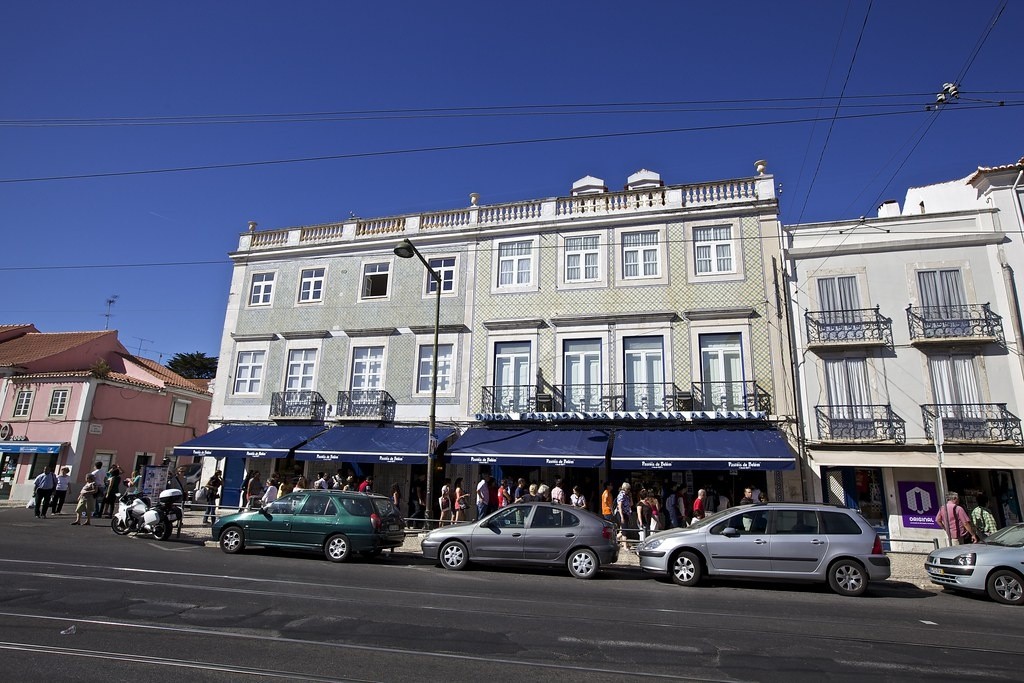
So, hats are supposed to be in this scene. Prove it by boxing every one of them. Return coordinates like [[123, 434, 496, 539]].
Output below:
[[62, 467, 69, 472], [346, 475, 354, 482]]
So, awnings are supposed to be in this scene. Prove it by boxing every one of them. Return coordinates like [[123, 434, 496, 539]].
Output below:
[[0, 441, 69, 453], [174, 425, 329, 459], [294, 426, 456, 465], [611, 428, 797, 471], [444, 428, 611, 468]]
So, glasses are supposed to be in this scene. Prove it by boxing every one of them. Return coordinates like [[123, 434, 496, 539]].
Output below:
[[179, 471, 185, 473]]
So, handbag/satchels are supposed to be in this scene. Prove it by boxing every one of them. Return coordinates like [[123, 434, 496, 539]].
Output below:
[[51, 473, 56, 491], [182, 492, 187, 501], [93, 486, 104, 498], [438, 496, 451, 511], [958, 530, 980, 545], [456, 488, 470, 509], [195, 486, 209, 501]]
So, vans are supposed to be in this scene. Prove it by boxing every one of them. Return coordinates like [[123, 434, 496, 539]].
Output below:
[[168, 462, 202, 508]]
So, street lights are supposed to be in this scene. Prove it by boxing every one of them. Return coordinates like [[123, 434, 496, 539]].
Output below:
[[392, 236, 444, 532]]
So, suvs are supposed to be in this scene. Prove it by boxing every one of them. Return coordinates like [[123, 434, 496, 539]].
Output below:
[[211, 489, 406, 563]]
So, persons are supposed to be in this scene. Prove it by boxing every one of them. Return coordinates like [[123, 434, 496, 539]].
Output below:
[[4, 458, 14, 469], [972, 491, 997, 539], [935, 492, 977, 546], [31, 462, 765, 551]]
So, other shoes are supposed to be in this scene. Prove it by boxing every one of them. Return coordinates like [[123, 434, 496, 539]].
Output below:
[[406, 520, 410, 529], [204, 522, 212, 525], [41, 515, 46, 519], [70, 521, 80, 525], [26, 496, 36, 509], [413, 526, 422, 529], [104, 512, 109, 515], [83, 522, 90, 525], [51, 513, 55, 516], [35, 516, 39, 518], [56, 512, 60, 514], [105, 516, 113, 518]]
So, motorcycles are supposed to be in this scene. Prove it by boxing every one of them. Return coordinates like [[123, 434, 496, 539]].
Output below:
[[111, 475, 184, 541]]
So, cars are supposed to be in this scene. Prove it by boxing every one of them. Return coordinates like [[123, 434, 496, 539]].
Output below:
[[923, 520, 1024, 608], [639, 500, 892, 597], [422, 501, 622, 580]]
